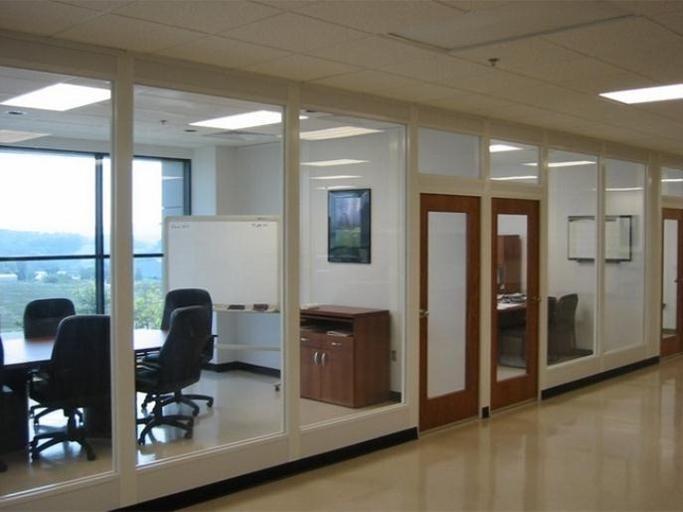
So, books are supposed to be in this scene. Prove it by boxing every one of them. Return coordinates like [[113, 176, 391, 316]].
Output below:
[[326, 329, 353, 337], [300, 322, 317, 328], [299, 327, 324, 332]]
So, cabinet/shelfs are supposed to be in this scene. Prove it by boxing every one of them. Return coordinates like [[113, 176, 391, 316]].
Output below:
[[299, 306, 392, 409]]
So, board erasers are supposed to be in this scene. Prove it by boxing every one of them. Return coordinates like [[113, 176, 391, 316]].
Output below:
[[253, 304, 268, 311], [228, 305, 244, 310]]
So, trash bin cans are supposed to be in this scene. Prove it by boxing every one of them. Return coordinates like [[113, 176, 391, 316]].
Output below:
[[0, 371, 29, 452]]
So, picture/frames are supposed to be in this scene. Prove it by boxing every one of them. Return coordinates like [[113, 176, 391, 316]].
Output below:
[[325, 187, 371, 265]]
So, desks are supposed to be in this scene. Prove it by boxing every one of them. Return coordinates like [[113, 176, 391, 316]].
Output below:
[[495, 298, 528, 362]]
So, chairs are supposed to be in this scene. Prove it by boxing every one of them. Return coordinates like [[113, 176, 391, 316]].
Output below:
[[548, 292, 581, 360], [0, 287, 215, 471]]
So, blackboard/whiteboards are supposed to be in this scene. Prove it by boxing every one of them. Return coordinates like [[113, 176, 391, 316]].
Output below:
[[163, 216, 283, 313]]
